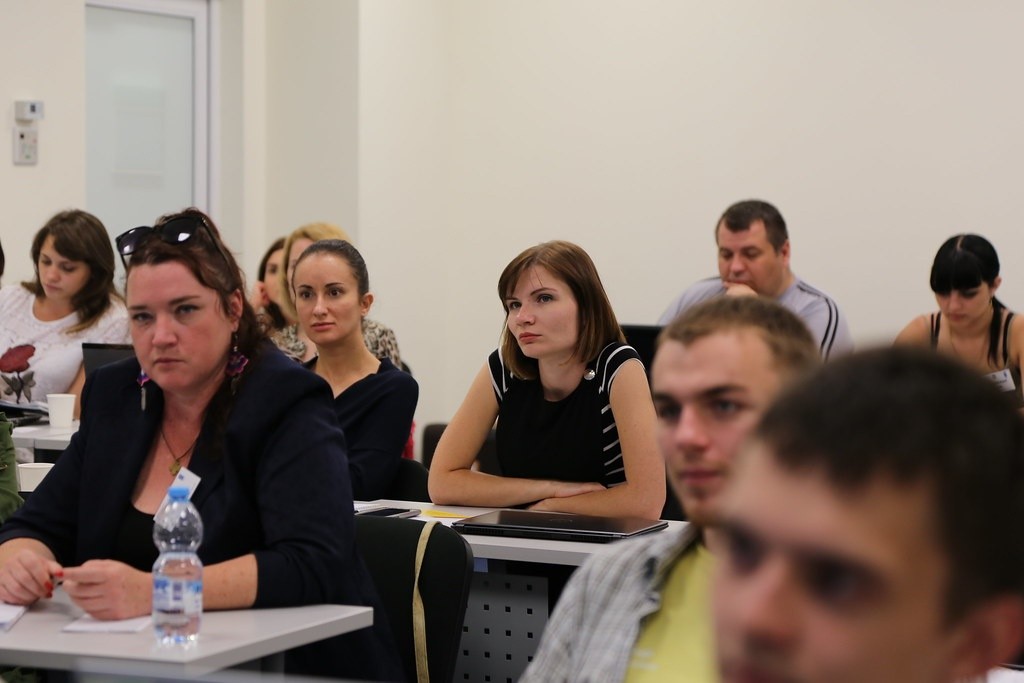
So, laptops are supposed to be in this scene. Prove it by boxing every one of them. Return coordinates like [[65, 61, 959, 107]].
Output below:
[[452, 509, 668, 540], [82, 342, 136, 380], [619, 324, 666, 386]]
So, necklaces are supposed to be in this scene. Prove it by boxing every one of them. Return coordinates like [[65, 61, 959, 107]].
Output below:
[[947, 328, 988, 372], [160, 430, 199, 476]]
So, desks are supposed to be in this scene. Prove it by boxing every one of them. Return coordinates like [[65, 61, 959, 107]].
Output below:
[[0, 409, 685, 683]]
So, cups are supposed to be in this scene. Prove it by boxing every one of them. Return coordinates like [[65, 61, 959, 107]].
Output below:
[[47, 393, 76, 431], [17, 463, 54, 491]]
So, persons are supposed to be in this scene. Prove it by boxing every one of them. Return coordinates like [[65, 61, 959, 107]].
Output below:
[[247, 224, 420, 513], [716, 344, 1024, 683], [659, 200, 852, 382], [0, 209, 132, 502], [428, 242, 666, 619], [0, 207, 406, 683], [892, 234, 1024, 410], [516, 297, 829, 683]]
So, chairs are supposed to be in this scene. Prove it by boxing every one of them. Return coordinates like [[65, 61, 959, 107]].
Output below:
[[277, 518, 471, 683], [414, 424, 448, 467]]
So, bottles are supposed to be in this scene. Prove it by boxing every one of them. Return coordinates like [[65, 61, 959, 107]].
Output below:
[[153, 487, 203, 647]]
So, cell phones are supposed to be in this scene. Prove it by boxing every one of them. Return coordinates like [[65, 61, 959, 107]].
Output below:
[[354, 508, 421, 519]]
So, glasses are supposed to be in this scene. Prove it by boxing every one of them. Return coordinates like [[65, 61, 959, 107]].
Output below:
[[116, 214, 233, 282]]
